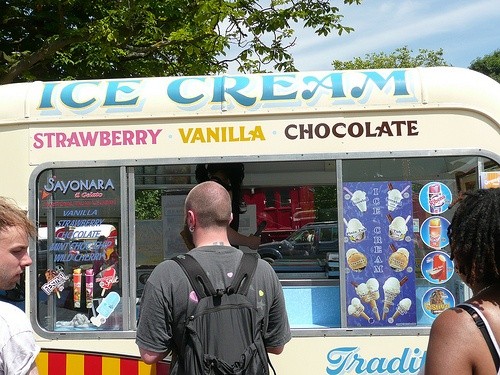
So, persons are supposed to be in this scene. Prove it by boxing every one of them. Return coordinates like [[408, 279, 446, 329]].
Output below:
[[91, 235, 119, 276], [184, 162, 261, 251], [306, 231, 314, 242], [135, 180, 292, 375], [425, 188, 500, 375], [0, 196, 41, 375]]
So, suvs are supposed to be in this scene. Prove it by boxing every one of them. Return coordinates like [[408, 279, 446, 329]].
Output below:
[[259, 221, 340, 271]]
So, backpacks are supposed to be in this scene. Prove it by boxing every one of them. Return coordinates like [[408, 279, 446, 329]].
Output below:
[[169, 253, 277, 375]]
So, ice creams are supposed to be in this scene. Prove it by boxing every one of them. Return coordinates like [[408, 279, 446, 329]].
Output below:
[[344, 189, 412, 323]]
